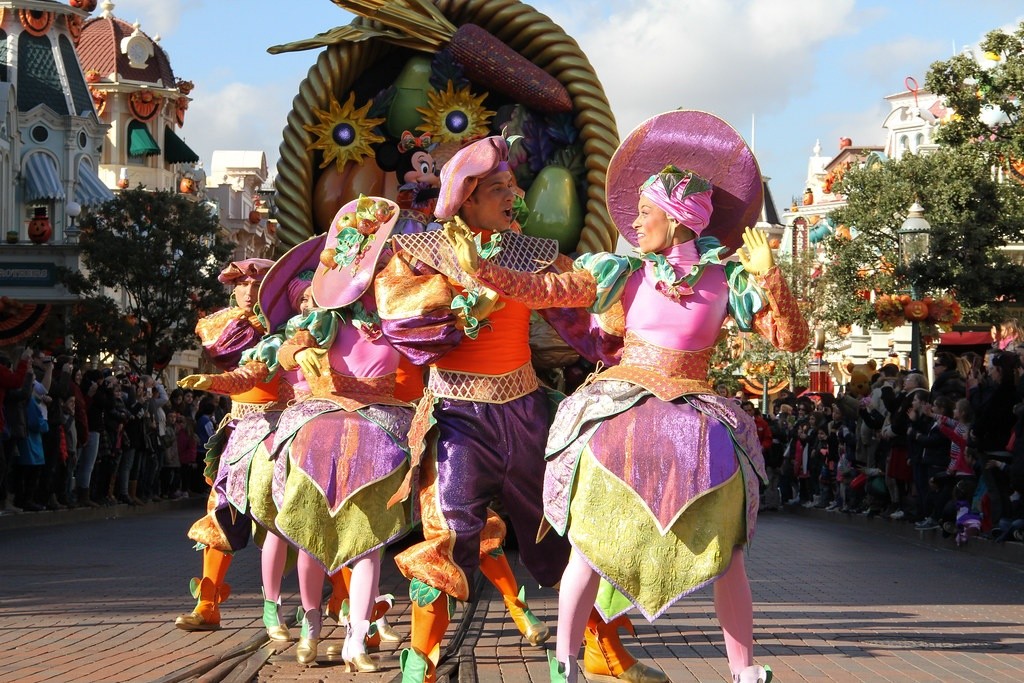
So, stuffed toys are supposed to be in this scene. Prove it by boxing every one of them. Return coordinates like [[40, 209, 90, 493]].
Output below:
[[375, 127, 442, 242]]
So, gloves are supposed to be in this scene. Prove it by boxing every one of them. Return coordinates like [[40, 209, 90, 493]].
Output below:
[[465, 288, 507, 324], [295, 348, 328, 377], [178, 374, 214, 390], [738, 227, 777, 280], [442, 214, 480, 276]]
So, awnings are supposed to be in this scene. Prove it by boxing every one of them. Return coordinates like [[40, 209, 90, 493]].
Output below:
[[164, 125, 201, 166], [124, 118, 162, 159], [23, 148, 68, 205], [71, 157, 121, 210]]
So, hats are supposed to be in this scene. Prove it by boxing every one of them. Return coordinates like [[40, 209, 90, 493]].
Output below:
[[434, 133, 510, 222], [902, 368, 923, 376], [218, 257, 276, 286], [32, 207, 49, 221]]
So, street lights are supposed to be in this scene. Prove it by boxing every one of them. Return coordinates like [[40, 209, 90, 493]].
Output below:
[[897, 197, 935, 369]]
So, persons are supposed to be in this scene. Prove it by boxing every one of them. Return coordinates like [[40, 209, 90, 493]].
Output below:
[[167, 253, 361, 633], [368, 136, 678, 683], [0, 341, 234, 510], [714, 340, 1024, 554], [273, 244, 412, 680], [357, 502, 559, 654], [439, 161, 814, 683], [180, 251, 412, 647]]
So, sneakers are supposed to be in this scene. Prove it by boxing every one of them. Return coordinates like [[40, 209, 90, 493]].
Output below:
[[914, 517, 941, 530]]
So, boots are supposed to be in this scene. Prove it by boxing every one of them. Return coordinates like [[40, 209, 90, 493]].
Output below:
[[296, 606, 323, 664], [261, 586, 292, 642], [342, 616, 379, 673], [374, 592, 402, 642], [175, 576, 231, 631], [725, 662, 773, 682], [502, 586, 551, 646], [547, 648, 578, 683], [399, 641, 442, 683], [326, 600, 381, 662], [582, 612, 669, 682]]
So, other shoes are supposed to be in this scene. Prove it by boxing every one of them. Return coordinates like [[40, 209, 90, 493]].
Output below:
[[1, 455, 210, 514], [943, 520, 957, 532], [787, 492, 906, 522]]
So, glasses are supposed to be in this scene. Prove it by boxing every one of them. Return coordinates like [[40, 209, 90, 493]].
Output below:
[[798, 407, 806, 412], [935, 362, 942, 368]]
